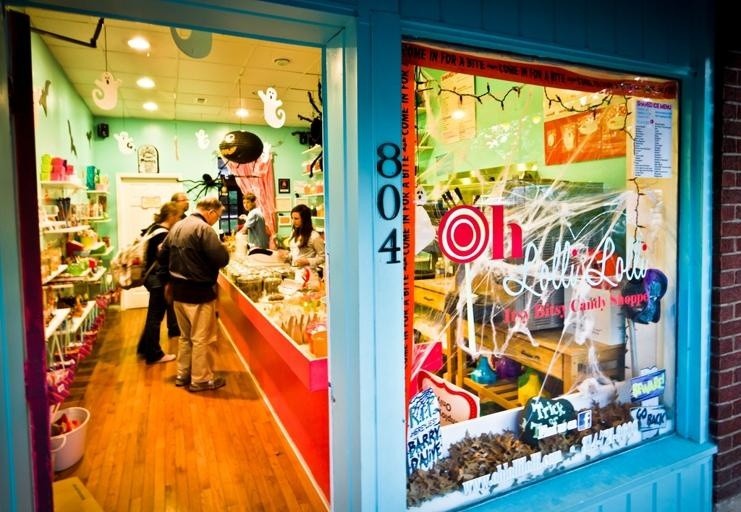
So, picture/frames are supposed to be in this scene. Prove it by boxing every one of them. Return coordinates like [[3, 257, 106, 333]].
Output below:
[[275, 179, 308, 227]]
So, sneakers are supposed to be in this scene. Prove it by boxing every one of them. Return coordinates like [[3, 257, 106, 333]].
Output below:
[[156, 353, 176, 363], [175, 375, 225, 394]]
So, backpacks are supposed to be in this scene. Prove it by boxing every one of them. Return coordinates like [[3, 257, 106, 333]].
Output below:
[[113, 224, 169, 290]]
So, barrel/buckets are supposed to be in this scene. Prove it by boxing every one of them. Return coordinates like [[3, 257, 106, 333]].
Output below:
[[48, 408, 91, 473]]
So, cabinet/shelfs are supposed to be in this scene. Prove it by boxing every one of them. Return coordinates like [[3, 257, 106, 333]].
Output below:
[[36, 183, 114, 416], [302, 145, 324, 233]]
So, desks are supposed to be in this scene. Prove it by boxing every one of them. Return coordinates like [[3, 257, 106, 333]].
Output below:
[[414, 275, 514, 383], [453, 320, 625, 410]]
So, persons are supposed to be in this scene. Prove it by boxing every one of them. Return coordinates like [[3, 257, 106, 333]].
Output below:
[[157, 196, 227, 393], [236, 191, 269, 251], [278, 203, 326, 273], [135, 201, 184, 366], [166, 191, 190, 340]]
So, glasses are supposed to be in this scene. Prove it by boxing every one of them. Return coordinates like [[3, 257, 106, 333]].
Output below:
[[208, 208, 221, 221]]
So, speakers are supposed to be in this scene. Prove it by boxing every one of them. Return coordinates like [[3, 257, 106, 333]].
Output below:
[[98, 123, 109, 137]]
